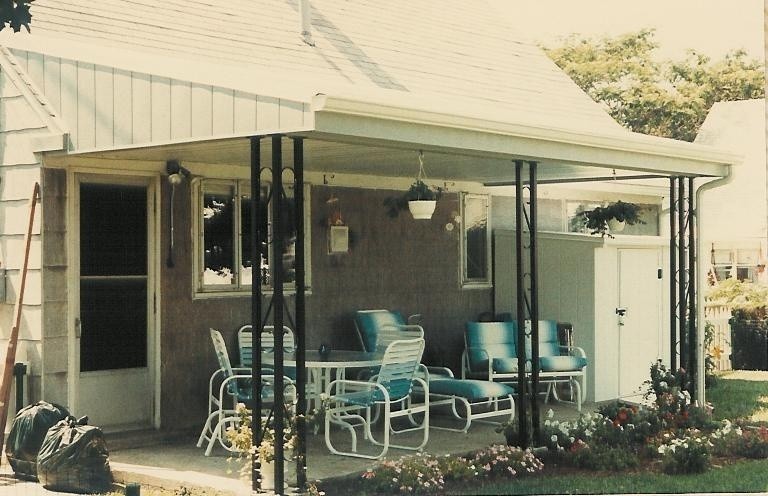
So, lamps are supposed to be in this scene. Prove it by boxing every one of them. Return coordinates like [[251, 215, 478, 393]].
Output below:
[[159, 159, 190, 187]]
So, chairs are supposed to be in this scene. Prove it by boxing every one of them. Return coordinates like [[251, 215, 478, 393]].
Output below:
[[315, 336, 428, 460], [232, 324, 315, 415], [352, 309, 515, 434], [339, 326, 425, 422], [195, 329, 295, 458]]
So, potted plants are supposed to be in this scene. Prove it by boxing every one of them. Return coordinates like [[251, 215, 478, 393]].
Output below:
[[376, 179, 450, 222], [571, 196, 650, 242]]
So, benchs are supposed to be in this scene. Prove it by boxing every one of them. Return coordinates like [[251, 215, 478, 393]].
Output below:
[[465, 320, 587, 411]]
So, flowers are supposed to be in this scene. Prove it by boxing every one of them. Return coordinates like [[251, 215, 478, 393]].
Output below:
[[217, 396, 323, 483]]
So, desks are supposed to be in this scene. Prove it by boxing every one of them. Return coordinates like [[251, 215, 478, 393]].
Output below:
[[257, 345, 386, 453]]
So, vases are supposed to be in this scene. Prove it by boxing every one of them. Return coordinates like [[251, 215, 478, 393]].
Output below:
[[260, 454, 290, 491]]
